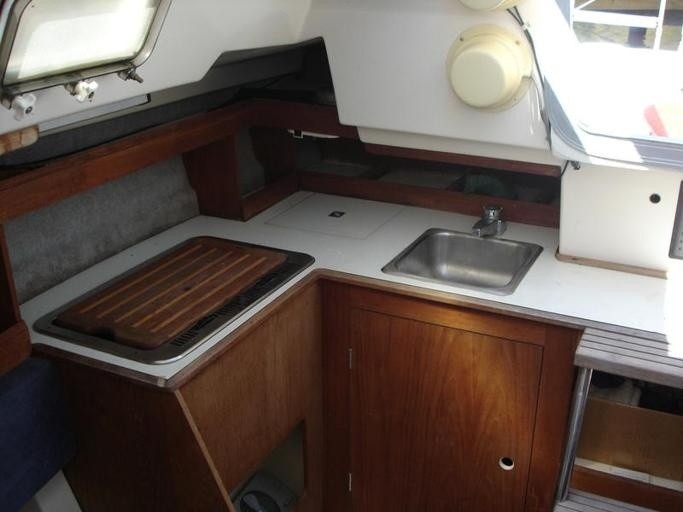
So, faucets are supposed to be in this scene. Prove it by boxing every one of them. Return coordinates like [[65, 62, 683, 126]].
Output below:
[[470, 204, 507, 239]]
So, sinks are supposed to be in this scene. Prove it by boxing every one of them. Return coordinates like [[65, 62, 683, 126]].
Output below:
[[379, 226, 544, 295]]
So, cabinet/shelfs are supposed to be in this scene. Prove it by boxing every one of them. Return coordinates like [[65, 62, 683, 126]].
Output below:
[[32, 277, 682, 511]]
